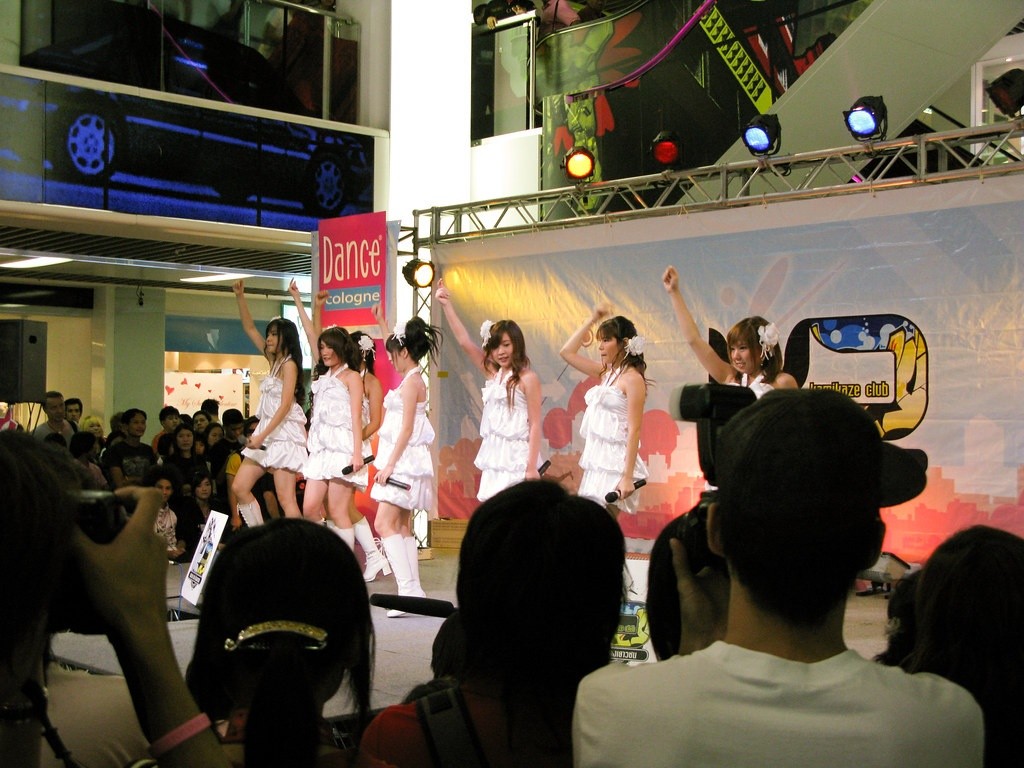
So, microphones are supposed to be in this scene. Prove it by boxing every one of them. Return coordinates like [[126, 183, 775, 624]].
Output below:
[[538, 460, 552, 475], [237, 435, 266, 451], [605, 480, 648, 502], [342, 455, 375, 475], [375, 477, 411, 491]]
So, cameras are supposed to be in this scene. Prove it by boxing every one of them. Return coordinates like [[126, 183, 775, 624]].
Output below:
[[668, 382, 757, 575], [65, 490, 129, 636]]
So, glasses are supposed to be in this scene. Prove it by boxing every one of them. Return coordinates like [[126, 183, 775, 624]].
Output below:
[[247, 429, 254, 434]]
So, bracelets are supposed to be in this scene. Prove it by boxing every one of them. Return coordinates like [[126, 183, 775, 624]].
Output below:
[[146, 713, 211, 757]]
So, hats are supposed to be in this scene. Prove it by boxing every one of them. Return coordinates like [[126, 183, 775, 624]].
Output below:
[[717, 388, 926, 523]]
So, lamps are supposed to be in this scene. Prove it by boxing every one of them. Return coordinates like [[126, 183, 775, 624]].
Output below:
[[984, 69, 1024, 119], [402, 258, 434, 291], [560, 144, 597, 186], [649, 131, 684, 171], [856, 551, 911, 600], [740, 114, 782, 158], [842, 94, 888, 143]]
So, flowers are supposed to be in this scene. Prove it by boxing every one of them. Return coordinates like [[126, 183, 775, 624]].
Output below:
[[269, 315, 781, 363]]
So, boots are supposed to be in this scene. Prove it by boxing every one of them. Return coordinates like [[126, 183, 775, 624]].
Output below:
[[353, 516, 392, 582], [337, 528, 355, 551], [238, 499, 264, 528], [403, 536, 426, 599], [382, 534, 417, 617]]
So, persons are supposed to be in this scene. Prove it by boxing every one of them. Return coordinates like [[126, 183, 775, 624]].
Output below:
[[559, 303, 650, 515], [0, 430, 230, 768], [571, 387, 984, 768], [35, 391, 260, 559], [881, 525, 1024, 768], [435, 287, 544, 502], [662, 265, 798, 400], [289, 277, 392, 581], [232, 280, 309, 526], [370, 303, 436, 618], [185, 520, 377, 768], [359, 479, 626, 768]]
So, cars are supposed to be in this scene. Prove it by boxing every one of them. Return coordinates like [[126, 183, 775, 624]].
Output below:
[[20, 16, 373, 220]]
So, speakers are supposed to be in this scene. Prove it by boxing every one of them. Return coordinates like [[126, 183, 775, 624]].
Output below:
[[0, 318, 48, 405]]
